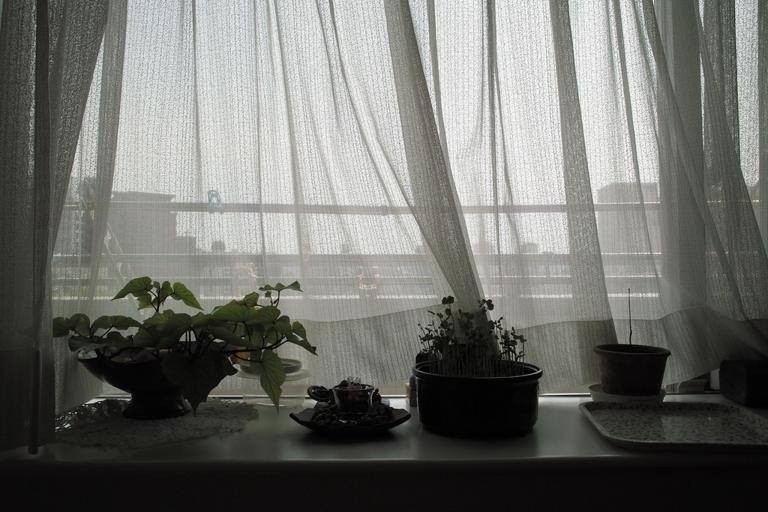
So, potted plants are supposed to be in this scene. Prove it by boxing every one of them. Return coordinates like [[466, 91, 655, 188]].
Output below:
[[52, 277, 320, 420], [410, 294, 543, 438]]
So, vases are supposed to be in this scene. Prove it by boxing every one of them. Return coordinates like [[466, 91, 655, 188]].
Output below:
[[592, 343, 672, 395]]
